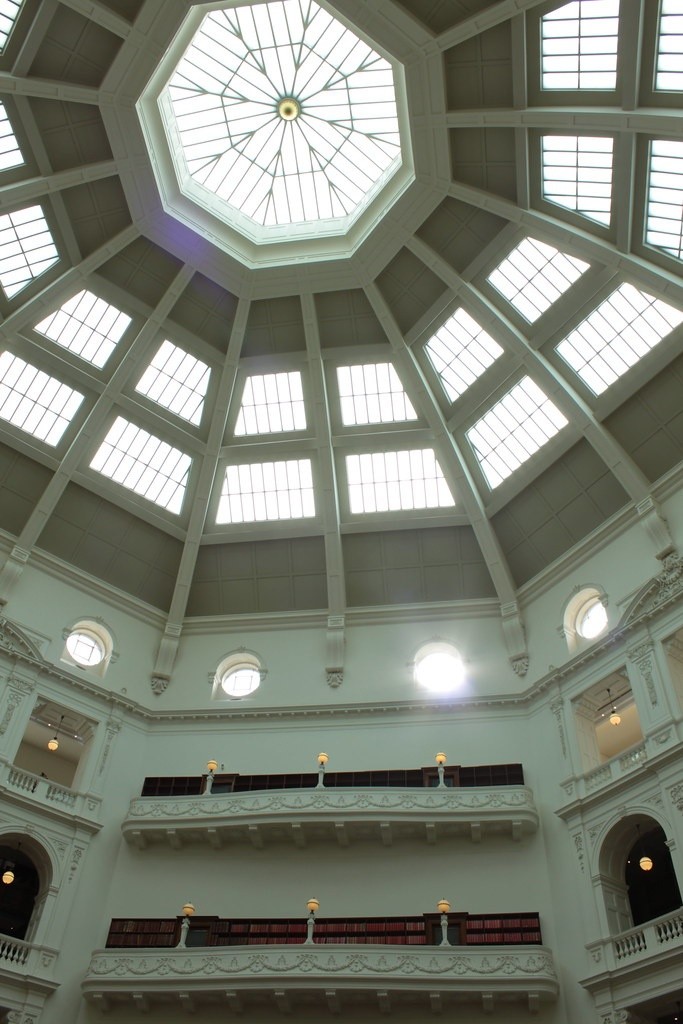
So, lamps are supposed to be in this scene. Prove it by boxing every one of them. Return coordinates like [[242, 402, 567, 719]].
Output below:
[[435, 752, 447, 764], [48, 715, 64, 750], [607, 689, 621, 725], [317, 752, 328, 765], [208, 759, 217, 773], [2, 842, 21, 884], [183, 902, 196, 915], [636, 824, 653, 870], [437, 898, 450, 912], [307, 897, 319, 912]]
